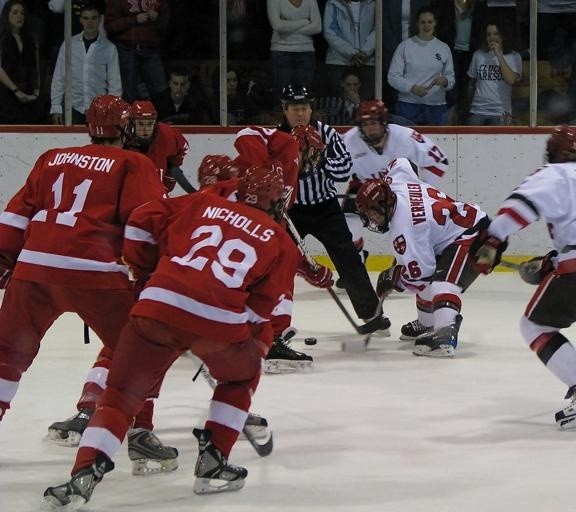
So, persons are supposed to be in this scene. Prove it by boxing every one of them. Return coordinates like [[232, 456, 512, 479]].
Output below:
[[1, 1, 271, 126], [354, 158, 508, 349], [0, 94, 179, 461], [230, 123, 334, 361], [124, 99, 190, 196], [46, 154, 271, 440], [44, 163, 302, 502], [468, 126, 576, 426], [338, 99, 450, 289], [267, 0, 576, 127], [275, 82, 390, 330]]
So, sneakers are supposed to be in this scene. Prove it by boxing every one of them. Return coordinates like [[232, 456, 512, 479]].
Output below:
[[48, 406, 93, 434], [194, 445, 248, 481], [127, 428, 178, 461], [415, 325, 458, 350], [43, 464, 103, 505], [401, 320, 434, 337], [264, 338, 313, 361], [554, 399, 575, 423]]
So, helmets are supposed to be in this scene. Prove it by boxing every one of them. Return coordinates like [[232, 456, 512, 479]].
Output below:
[[129, 100, 157, 143], [356, 100, 388, 145], [281, 86, 315, 105], [237, 168, 285, 223], [292, 125, 326, 180], [356, 180, 394, 233], [546, 126, 576, 162], [89, 94, 130, 139], [199, 155, 232, 185]]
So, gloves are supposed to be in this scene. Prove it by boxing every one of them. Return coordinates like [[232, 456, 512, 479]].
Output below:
[[519, 256, 553, 284], [300, 263, 335, 288], [472, 236, 503, 275], [376, 265, 405, 298]]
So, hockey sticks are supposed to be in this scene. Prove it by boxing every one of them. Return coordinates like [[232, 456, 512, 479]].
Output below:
[[342, 259, 396, 351], [187, 349, 272, 457], [282, 211, 391, 334]]
